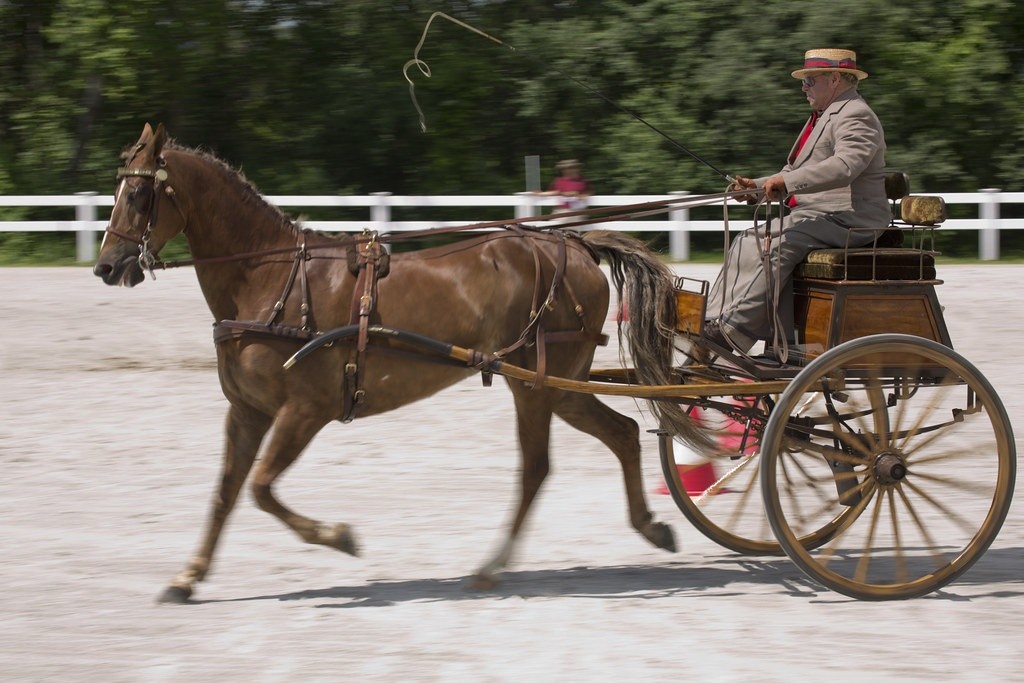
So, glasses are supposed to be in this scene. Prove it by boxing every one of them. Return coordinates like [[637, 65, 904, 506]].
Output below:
[[802, 74, 827, 87]]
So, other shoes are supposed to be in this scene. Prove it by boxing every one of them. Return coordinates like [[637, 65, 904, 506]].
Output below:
[[703, 318, 734, 353]]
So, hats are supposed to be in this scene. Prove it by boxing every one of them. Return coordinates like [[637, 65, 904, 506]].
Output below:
[[790, 48, 868, 81]]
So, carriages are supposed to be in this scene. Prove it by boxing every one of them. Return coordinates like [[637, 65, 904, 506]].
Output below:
[[95, 120, 1017, 605]]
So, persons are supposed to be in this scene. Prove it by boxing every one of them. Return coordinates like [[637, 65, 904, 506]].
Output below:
[[544, 159, 588, 236], [704, 48, 892, 355]]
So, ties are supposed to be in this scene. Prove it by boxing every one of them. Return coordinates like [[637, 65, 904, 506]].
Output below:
[[788, 112, 821, 209]]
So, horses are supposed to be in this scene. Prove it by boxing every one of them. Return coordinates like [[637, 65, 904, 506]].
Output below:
[[92, 119, 745, 607]]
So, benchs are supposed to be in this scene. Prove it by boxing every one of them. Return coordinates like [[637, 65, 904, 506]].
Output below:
[[764, 170, 955, 366]]
